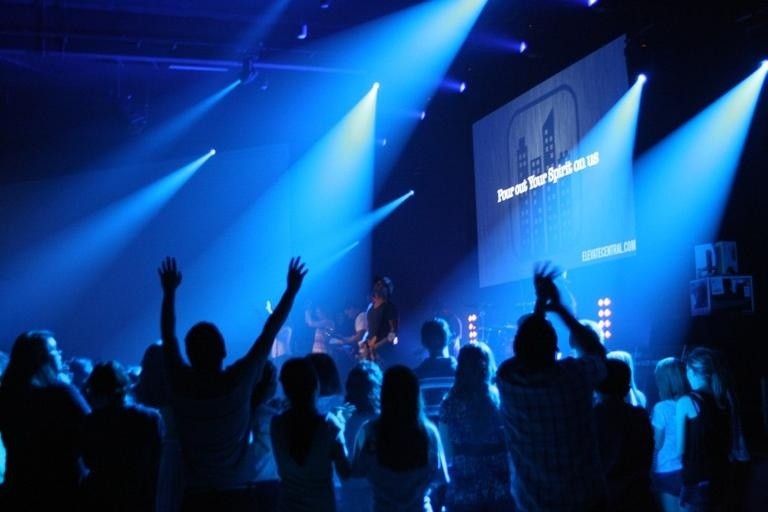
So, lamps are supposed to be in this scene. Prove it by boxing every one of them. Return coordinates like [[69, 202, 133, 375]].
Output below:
[[240, 68, 259, 86], [297, 24, 308, 39]]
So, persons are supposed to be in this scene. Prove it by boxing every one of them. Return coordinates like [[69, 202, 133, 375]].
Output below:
[[0, 257, 767, 512]]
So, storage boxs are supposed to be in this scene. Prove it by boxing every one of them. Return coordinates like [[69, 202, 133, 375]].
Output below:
[[689, 276, 754, 317], [692, 240, 739, 279]]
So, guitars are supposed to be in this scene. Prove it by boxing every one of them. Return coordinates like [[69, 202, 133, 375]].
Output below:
[[359, 333, 394, 362], [324, 326, 360, 361]]
